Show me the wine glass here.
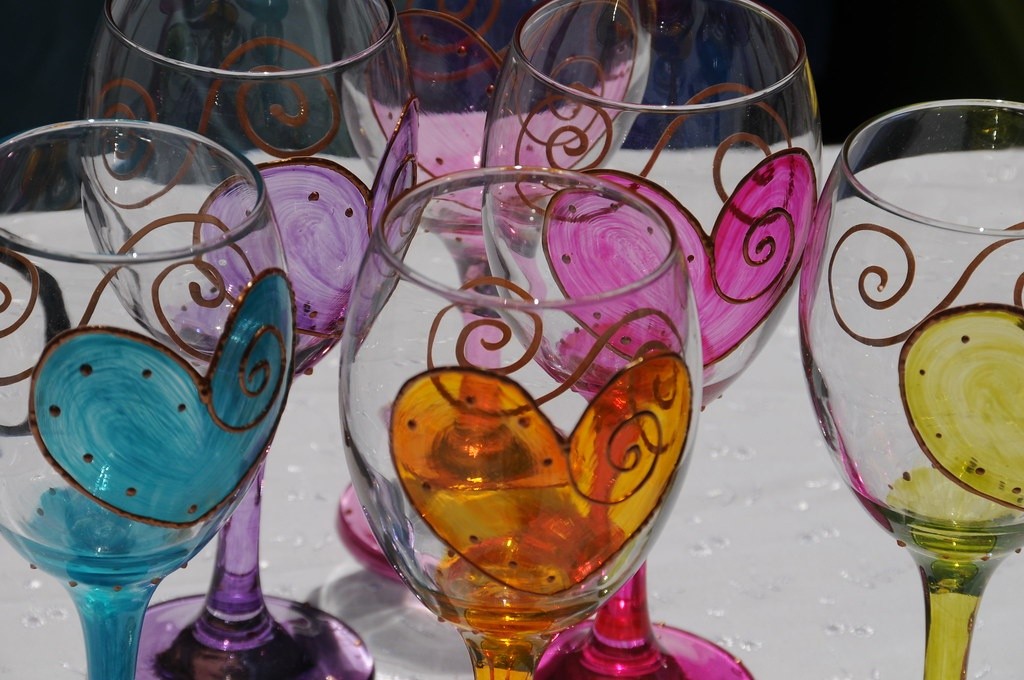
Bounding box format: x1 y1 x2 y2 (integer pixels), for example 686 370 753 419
0 119 295 680
798 101 1024 680
340 167 704 680
343 0 651 586
481 0 822 680
85 0 421 680
